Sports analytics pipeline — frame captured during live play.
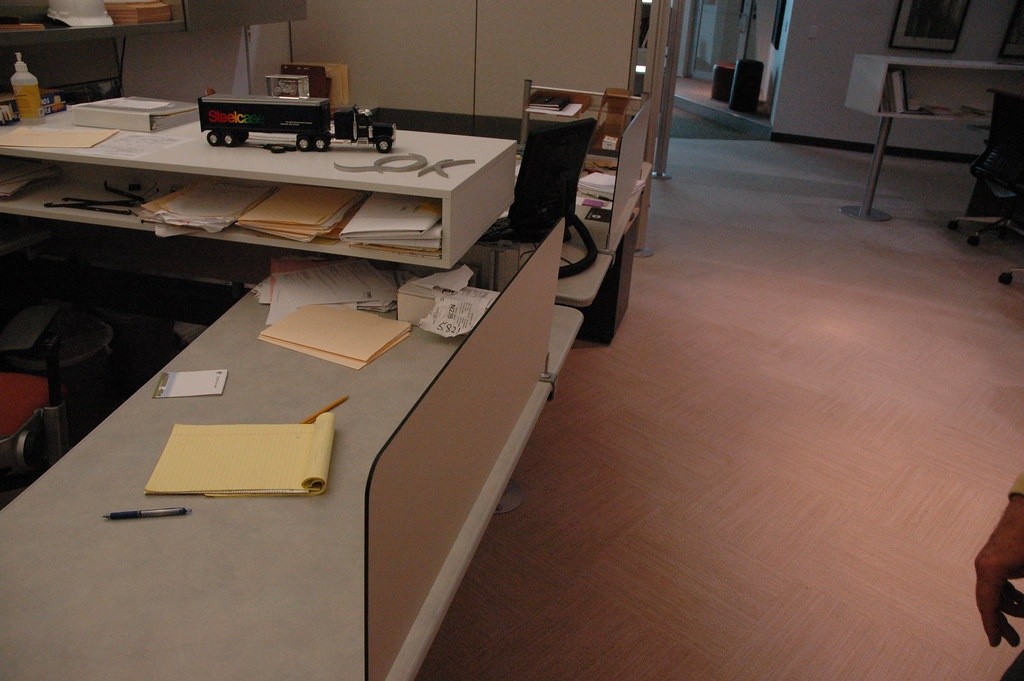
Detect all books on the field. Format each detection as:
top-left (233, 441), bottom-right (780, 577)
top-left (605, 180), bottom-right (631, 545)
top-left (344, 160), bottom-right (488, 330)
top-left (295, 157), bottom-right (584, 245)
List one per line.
top-left (529, 95), bottom-right (570, 110)
top-left (144, 411), bottom-right (335, 497)
top-left (880, 69), bottom-right (909, 113)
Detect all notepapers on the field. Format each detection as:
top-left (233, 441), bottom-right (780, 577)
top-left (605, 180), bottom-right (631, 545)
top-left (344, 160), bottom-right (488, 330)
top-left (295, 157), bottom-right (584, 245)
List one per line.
top-left (582, 199), bottom-right (603, 208)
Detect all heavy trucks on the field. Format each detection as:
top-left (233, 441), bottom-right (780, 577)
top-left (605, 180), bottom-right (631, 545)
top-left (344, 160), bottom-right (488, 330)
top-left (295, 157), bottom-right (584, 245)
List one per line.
top-left (197, 93), bottom-right (398, 152)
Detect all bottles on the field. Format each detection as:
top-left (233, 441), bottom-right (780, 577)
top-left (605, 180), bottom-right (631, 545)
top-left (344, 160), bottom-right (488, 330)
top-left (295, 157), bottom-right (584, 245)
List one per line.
top-left (11, 52), bottom-right (47, 126)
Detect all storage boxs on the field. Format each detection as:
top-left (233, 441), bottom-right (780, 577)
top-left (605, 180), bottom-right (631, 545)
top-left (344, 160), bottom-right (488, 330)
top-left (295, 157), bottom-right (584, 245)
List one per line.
top-left (398, 277), bottom-right (437, 325)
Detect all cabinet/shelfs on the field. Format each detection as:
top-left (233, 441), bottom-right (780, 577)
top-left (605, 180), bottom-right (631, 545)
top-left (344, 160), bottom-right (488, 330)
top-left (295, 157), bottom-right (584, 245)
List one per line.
top-left (0, 0), bottom-right (308, 48)
top-left (0, 101), bottom-right (514, 269)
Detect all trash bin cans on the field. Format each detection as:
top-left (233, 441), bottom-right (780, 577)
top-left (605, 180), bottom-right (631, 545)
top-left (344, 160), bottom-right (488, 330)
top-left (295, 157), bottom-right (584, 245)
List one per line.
top-left (728, 58), bottom-right (764, 113)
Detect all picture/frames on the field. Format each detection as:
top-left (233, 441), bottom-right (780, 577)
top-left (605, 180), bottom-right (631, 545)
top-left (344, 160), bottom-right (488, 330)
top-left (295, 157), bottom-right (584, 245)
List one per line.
top-left (770, 0), bottom-right (787, 49)
top-left (999, 0), bottom-right (1024, 57)
top-left (889, 0), bottom-right (970, 53)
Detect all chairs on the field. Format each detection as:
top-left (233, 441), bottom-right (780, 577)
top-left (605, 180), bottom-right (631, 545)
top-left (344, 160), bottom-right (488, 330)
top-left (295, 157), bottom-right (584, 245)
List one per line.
top-left (948, 89), bottom-right (1024, 245)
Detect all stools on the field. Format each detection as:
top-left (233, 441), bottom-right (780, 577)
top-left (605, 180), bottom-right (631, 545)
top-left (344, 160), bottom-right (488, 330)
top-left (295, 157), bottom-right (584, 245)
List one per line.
top-left (1, 311), bottom-right (130, 468)
top-left (0, 372), bottom-right (68, 475)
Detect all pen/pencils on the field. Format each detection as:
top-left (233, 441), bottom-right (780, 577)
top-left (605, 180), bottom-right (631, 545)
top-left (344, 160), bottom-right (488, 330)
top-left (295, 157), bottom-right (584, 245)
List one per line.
top-left (302, 393), bottom-right (350, 423)
top-left (581, 191), bottom-right (613, 202)
top-left (102, 507), bottom-right (192, 519)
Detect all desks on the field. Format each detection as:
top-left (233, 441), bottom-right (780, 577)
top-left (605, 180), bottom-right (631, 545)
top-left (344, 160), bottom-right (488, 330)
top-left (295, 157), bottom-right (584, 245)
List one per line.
top-left (839, 54), bottom-right (1024, 222)
top-left (0, 78), bottom-right (651, 681)
